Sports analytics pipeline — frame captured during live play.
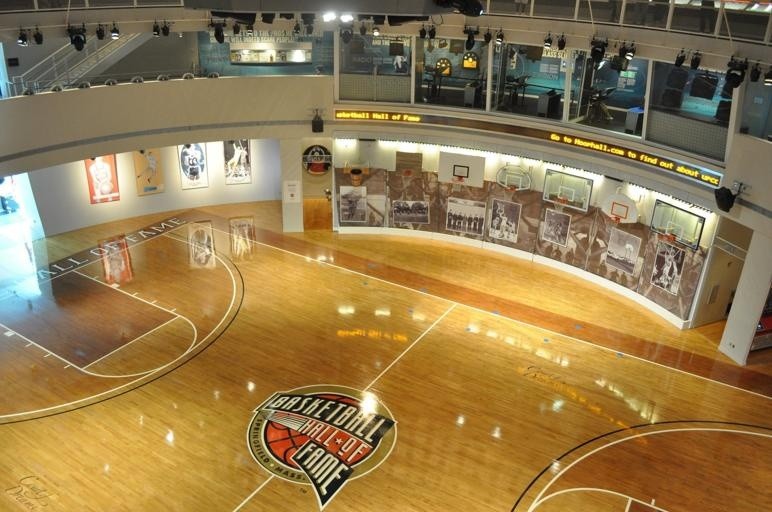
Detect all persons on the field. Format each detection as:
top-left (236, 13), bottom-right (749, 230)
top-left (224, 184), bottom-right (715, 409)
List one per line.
top-left (238, 226), bottom-right (253, 260)
top-left (88, 156), bottom-right (113, 202)
top-left (192, 230), bottom-right (211, 264)
top-left (226, 142), bottom-right (244, 178)
top-left (335, 171), bottom-right (704, 320)
top-left (102, 241), bottom-right (126, 284)
top-left (184, 143), bottom-right (202, 179)
top-left (238, 140), bottom-right (249, 177)
top-left (234, 227), bottom-right (244, 261)
top-left (137, 151), bottom-right (161, 185)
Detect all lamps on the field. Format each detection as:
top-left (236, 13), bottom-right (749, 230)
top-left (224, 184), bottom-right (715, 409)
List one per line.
top-left (260, 12), bottom-right (315, 36)
top-left (162, 20), bottom-right (171, 37)
top-left (94, 24), bottom-right (107, 42)
top-left (485, 28), bottom-right (492, 44)
top-left (543, 34), bottom-right (554, 49)
top-left (496, 27), bottom-right (504, 45)
top-left (151, 21), bottom-right (160, 37)
top-left (618, 40), bottom-right (637, 61)
top-left (33, 26), bottom-right (44, 45)
top-left (764, 66), bottom-right (772, 87)
top-left (556, 33), bottom-right (567, 51)
top-left (689, 50), bottom-right (703, 71)
top-left (389, 36), bottom-right (405, 58)
top-left (722, 55), bottom-right (747, 88)
top-left (463, 23), bottom-right (481, 51)
top-left (429, 24), bottom-right (437, 39)
top-left (749, 61), bottom-right (762, 82)
top-left (207, 10), bottom-right (257, 44)
top-left (323, 12), bottom-right (387, 45)
top-left (419, 21), bottom-right (427, 38)
top-left (66, 25), bottom-right (88, 52)
top-left (110, 22), bottom-right (121, 42)
top-left (688, 71), bottom-right (719, 101)
top-left (674, 48), bottom-right (690, 66)
top-left (18, 29), bottom-right (31, 48)
top-left (588, 35), bottom-right (609, 63)
